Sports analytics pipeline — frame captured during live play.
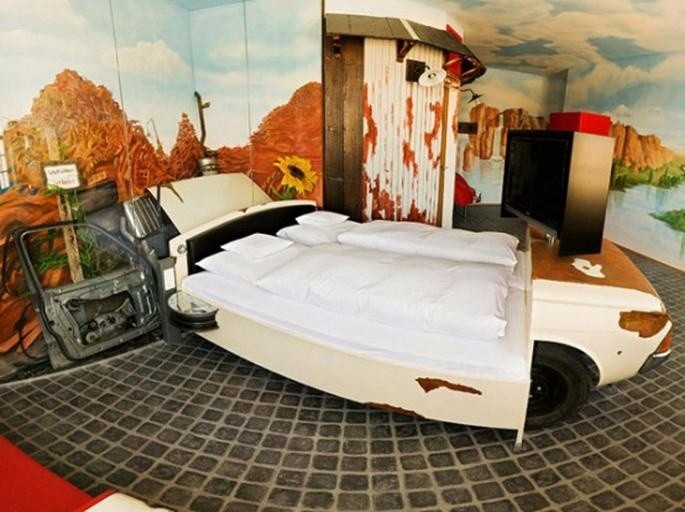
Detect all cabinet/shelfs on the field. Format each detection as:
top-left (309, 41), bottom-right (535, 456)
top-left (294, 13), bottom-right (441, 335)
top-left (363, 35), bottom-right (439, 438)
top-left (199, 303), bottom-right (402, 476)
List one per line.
top-left (498, 127), bottom-right (619, 260)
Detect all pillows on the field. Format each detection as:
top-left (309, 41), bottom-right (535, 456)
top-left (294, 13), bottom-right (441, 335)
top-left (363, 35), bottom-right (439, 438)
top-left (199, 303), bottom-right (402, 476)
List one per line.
top-left (295, 210), bottom-right (349, 227)
top-left (221, 232), bottom-right (293, 259)
top-left (276, 221), bottom-right (361, 248)
top-left (195, 240), bottom-right (310, 283)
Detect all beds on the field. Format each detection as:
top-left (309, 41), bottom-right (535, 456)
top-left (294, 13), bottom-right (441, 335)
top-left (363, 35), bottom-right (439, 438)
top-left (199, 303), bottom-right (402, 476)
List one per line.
top-left (168, 198), bottom-right (534, 452)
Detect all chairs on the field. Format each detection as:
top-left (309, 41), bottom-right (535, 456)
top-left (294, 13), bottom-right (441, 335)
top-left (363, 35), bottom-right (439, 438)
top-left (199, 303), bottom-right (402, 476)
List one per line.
top-left (454, 172), bottom-right (476, 217)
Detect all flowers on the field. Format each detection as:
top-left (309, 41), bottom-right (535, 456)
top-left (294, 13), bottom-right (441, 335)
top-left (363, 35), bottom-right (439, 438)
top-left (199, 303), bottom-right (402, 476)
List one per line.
top-left (270, 154), bottom-right (320, 200)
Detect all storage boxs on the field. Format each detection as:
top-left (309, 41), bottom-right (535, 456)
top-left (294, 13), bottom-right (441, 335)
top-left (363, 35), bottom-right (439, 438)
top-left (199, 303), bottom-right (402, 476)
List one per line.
top-left (549, 111), bottom-right (611, 138)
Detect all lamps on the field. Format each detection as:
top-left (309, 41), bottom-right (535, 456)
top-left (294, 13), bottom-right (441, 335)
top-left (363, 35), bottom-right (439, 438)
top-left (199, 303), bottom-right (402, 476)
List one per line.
top-left (453, 83), bottom-right (484, 105)
top-left (406, 58), bottom-right (447, 89)
